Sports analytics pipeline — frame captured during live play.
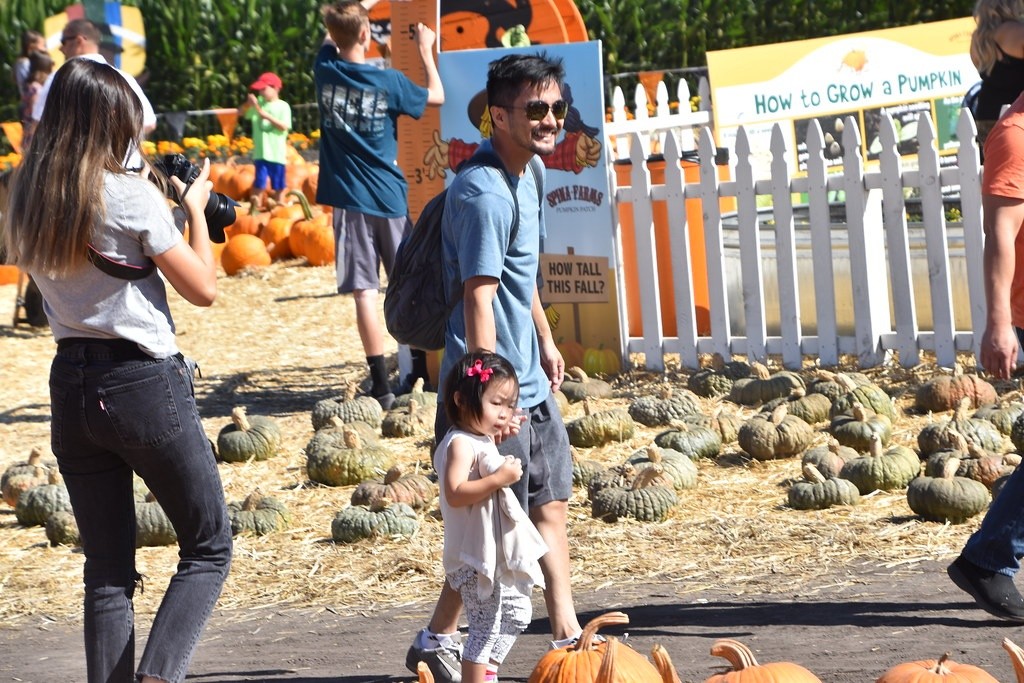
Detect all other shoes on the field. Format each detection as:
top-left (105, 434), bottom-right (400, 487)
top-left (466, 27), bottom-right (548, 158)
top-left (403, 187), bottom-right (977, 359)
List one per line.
top-left (367, 390), bottom-right (395, 411)
top-left (948, 557), bottom-right (1024, 623)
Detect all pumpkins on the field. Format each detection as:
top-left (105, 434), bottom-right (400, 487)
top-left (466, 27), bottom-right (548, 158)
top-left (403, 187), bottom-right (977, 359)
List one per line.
top-left (555, 361), bottom-right (1024, 522)
top-left (215, 376), bottom-right (439, 543)
top-left (0, 264), bottom-right (29, 286)
top-left (1, 447), bottom-right (178, 548)
top-left (526, 612), bottom-right (1024, 683)
top-left (163, 145), bottom-right (337, 275)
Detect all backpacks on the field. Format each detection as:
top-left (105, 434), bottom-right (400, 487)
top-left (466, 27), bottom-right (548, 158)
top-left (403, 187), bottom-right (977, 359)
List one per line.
top-left (384, 150), bottom-right (544, 351)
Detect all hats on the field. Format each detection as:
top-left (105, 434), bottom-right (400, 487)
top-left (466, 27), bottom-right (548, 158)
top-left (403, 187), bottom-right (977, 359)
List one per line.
top-left (251, 72), bottom-right (282, 90)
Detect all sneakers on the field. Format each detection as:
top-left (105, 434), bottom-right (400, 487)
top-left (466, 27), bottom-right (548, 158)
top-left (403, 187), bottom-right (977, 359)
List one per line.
top-left (405, 629), bottom-right (467, 683)
top-left (548, 630), bottom-right (648, 659)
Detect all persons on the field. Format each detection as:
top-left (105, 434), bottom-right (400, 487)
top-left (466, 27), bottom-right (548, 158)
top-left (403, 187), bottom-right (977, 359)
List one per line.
top-left (435, 347), bottom-right (532, 683)
top-left (239, 72), bottom-right (292, 205)
top-left (969, 0), bottom-right (1024, 140)
top-left (28, 19), bottom-right (156, 335)
top-left (17, 29), bottom-right (56, 153)
top-left (312, 5), bottom-right (445, 412)
top-left (402, 54), bottom-right (632, 683)
top-left (7, 59), bottom-right (232, 683)
top-left (945, 91), bottom-right (1023, 621)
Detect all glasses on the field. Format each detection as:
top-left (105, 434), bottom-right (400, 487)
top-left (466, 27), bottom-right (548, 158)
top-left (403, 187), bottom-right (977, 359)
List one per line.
top-left (60, 34), bottom-right (88, 42)
top-left (500, 98), bottom-right (569, 121)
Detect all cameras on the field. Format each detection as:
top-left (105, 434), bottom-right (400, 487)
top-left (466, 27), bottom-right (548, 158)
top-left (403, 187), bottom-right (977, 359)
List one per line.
top-left (146, 153), bottom-right (241, 244)
